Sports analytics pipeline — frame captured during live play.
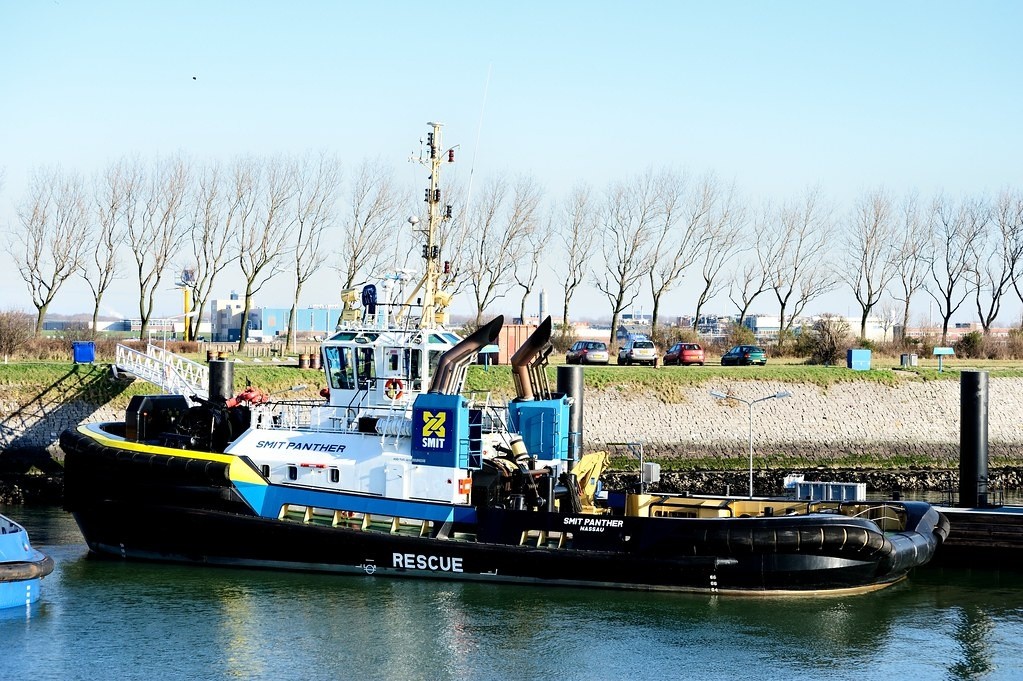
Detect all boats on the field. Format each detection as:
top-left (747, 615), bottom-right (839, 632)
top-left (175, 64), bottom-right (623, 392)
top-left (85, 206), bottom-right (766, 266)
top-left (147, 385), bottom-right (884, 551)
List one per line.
top-left (59, 119), bottom-right (951, 600)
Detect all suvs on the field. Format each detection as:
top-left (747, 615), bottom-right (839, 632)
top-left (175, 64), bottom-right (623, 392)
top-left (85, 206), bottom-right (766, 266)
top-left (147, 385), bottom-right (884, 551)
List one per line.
top-left (617, 339), bottom-right (659, 366)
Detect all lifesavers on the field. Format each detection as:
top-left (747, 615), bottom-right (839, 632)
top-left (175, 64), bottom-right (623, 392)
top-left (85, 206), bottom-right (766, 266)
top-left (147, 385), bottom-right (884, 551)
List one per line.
top-left (385, 379), bottom-right (405, 400)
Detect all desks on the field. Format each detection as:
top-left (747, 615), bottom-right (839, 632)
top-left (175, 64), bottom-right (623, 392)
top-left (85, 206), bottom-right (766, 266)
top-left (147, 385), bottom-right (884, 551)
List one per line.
top-left (796, 481), bottom-right (867, 502)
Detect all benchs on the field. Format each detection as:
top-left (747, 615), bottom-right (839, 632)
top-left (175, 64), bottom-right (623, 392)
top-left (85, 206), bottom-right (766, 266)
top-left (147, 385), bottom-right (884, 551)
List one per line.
top-left (940, 479), bottom-right (959, 507)
top-left (977, 480), bottom-right (1004, 509)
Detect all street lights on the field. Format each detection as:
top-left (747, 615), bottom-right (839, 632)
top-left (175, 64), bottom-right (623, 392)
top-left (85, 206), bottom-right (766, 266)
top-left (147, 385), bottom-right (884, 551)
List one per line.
top-left (708, 390), bottom-right (792, 501)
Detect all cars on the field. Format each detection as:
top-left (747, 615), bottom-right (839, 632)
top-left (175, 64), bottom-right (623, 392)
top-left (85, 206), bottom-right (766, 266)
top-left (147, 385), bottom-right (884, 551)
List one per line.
top-left (721, 345), bottom-right (767, 366)
top-left (566, 341), bottom-right (609, 365)
top-left (663, 343), bottom-right (705, 367)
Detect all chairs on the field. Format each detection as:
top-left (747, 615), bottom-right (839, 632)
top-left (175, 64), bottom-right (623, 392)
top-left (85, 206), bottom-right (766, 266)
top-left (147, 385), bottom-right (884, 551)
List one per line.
top-left (639, 344), bottom-right (643, 347)
